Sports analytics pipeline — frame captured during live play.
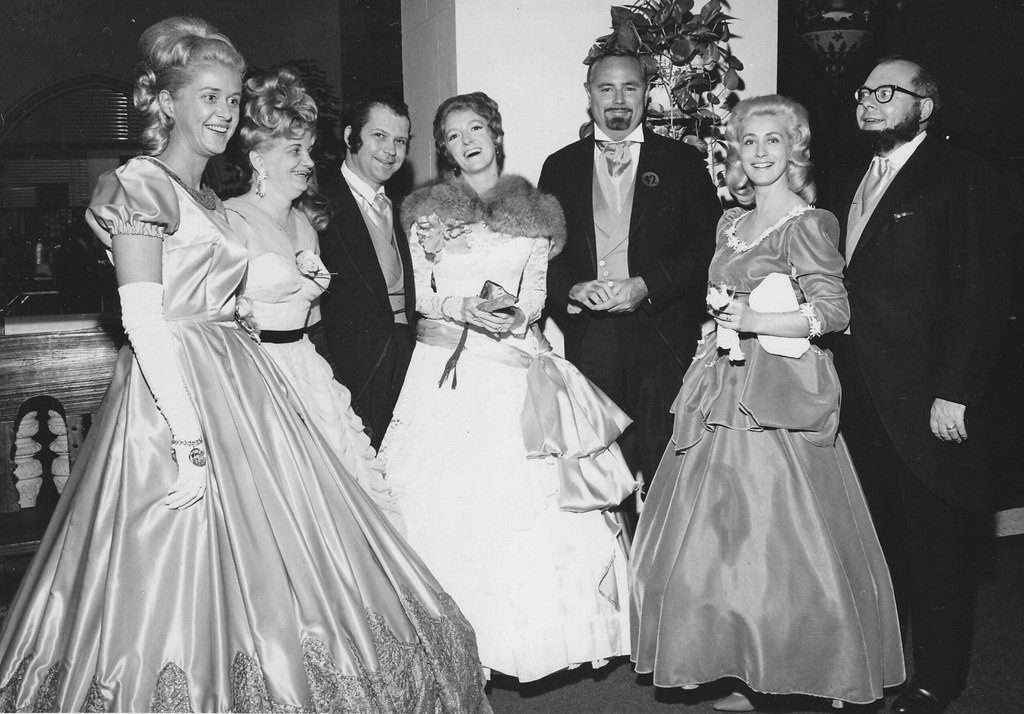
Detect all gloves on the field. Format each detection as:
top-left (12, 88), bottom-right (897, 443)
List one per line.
top-left (304, 319), bottom-right (372, 440)
top-left (118, 283), bottom-right (206, 510)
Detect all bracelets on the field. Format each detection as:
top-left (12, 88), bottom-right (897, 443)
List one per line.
top-left (170, 435), bottom-right (208, 468)
top-left (440, 295), bottom-right (456, 322)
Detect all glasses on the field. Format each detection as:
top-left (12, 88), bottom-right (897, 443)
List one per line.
top-left (855, 85), bottom-right (926, 105)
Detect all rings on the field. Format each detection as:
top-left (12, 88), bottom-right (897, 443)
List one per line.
top-left (472, 316), bottom-right (477, 321)
top-left (724, 315), bottom-right (731, 321)
top-left (947, 424), bottom-right (956, 430)
top-left (498, 324), bottom-right (503, 331)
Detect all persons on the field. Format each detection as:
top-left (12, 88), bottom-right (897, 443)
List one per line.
top-left (201, 65), bottom-right (409, 562)
top-left (293, 88), bottom-right (418, 451)
top-left (369, 91), bottom-right (636, 687)
top-left (823, 56), bottom-right (989, 714)
top-left (534, 50), bottom-right (720, 561)
top-left (81, 13), bottom-right (300, 714)
top-left (622, 94), bottom-right (907, 711)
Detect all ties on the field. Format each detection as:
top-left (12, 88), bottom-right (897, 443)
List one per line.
top-left (346, 179), bottom-right (392, 244)
top-left (862, 159), bottom-right (892, 215)
top-left (596, 140), bottom-right (635, 214)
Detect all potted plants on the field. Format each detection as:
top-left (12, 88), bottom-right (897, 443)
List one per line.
top-left (580, 0), bottom-right (743, 212)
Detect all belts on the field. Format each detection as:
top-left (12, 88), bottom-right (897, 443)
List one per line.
top-left (259, 328), bottom-right (304, 344)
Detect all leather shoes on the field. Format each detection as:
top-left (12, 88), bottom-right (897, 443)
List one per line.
top-left (891, 676), bottom-right (952, 714)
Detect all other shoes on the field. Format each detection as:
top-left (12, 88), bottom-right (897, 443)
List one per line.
top-left (714, 692), bottom-right (755, 712)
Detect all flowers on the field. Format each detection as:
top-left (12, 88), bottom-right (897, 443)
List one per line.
top-left (294, 249), bottom-right (336, 294)
top-left (414, 213), bottom-right (472, 265)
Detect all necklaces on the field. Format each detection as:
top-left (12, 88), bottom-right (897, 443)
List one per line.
top-left (479, 220), bottom-right (488, 228)
